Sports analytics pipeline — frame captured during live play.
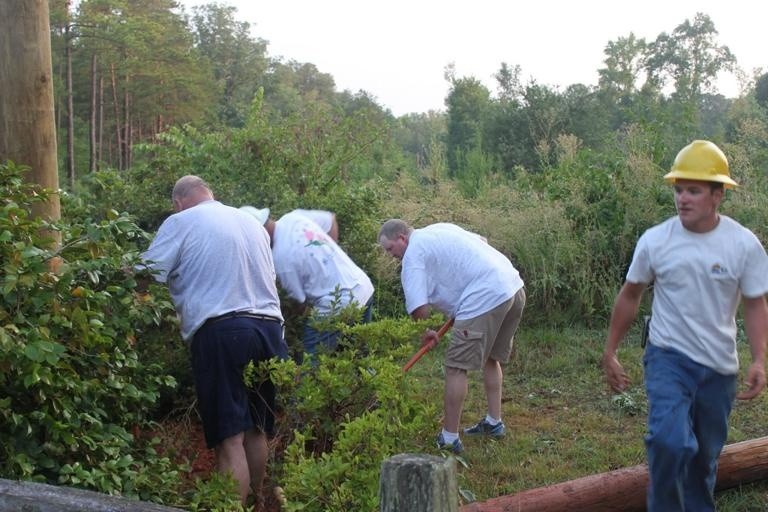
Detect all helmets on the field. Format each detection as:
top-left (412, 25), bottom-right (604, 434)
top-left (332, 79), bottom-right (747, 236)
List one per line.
top-left (663, 139), bottom-right (739, 190)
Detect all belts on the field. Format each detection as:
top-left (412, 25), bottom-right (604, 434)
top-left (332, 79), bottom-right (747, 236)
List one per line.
top-left (209, 310), bottom-right (281, 323)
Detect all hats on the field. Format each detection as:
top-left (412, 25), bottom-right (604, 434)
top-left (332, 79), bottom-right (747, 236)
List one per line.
top-left (239, 206), bottom-right (270, 225)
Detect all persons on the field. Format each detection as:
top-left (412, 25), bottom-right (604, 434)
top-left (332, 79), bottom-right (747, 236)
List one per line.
top-left (602, 140), bottom-right (767, 510)
top-left (377, 219), bottom-right (527, 452)
top-left (121, 174), bottom-right (290, 510)
top-left (239, 206), bottom-right (375, 451)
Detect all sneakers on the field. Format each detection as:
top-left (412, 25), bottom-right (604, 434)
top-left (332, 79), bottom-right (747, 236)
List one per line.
top-left (434, 432), bottom-right (463, 455)
top-left (464, 419), bottom-right (505, 437)
